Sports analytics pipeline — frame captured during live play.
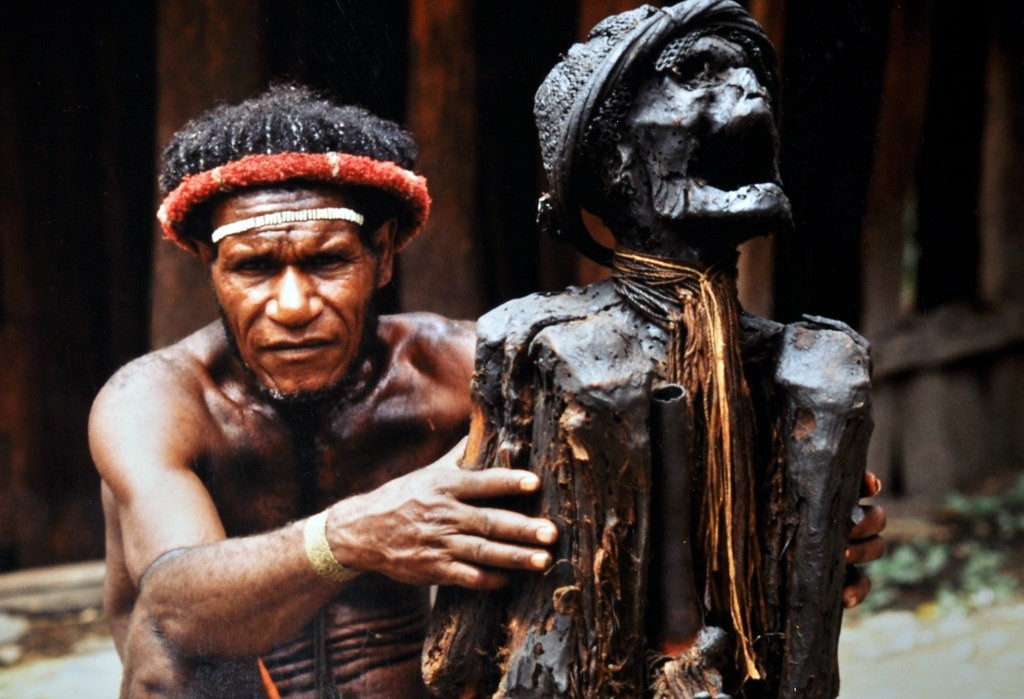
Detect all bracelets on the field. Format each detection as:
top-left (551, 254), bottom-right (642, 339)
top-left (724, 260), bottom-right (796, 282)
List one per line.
top-left (301, 504), bottom-right (359, 587)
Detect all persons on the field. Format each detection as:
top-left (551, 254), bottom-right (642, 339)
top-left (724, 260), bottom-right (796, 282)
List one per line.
top-left (86, 78), bottom-right (888, 699)
top-left (411, 0), bottom-right (877, 699)
